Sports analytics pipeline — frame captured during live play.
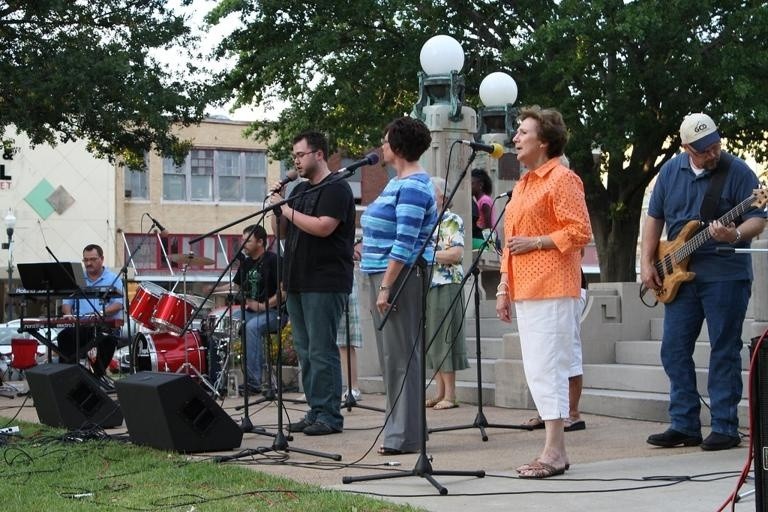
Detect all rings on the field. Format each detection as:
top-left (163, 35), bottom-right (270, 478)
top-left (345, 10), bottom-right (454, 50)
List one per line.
top-left (498, 315), bottom-right (501, 318)
top-left (714, 233), bottom-right (717, 236)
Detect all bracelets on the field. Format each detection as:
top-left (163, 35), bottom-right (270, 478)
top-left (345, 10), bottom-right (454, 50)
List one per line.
top-left (496, 282), bottom-right (510, 289)
top-left (731, 230), bottom-right (742, 246)
top-left (496, 292), bottom-right (508, 296)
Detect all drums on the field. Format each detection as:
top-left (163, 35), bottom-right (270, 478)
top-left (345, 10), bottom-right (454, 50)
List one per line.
top-left (129, 282), bottom-right (166, 331)
top-left (208, 315), bottom-right (237, 337)
top-left (149, 291), bottom-right (192, 333)
top-left (131, 332), bottom-right (205, 374)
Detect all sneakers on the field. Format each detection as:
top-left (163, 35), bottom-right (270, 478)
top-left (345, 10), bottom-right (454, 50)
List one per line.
top-left (302, 421), bottom-right (343, 436)
top-left (285, 419), bottom-right (315, 432)
top-left (342, 389), bottom-right (361, 401)
top-left (236, 383), bottom-right (263, 396)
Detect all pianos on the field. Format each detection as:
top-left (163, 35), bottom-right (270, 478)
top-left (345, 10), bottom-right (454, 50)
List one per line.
top-left (8, 286), bottom-right (123, 300)
top-left (22, 317), bottom-right (123, 331)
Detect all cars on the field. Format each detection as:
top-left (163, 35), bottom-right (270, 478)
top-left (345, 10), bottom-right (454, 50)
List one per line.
top-left (0, 320), bottom-right (60, 380)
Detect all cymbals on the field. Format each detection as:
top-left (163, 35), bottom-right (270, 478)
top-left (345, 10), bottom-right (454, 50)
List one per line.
top-left (166, 252), bottom-right (214, 268)
top-left (214, 281), bottom-right (244, 293)
top-left (180, 292), bottom-right (213, 311)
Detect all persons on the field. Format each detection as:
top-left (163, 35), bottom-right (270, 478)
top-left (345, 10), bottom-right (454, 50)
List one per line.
top-left (360, 117), bottom-right (437, 456)
top-left (58, 244), bottom-right (126, 379)
top-left (640, 113), bottom-right (767, 450)
top-left (202, 224), bottom-right (289, 394)
top-left (268, 132), bottom-right (355, 435)
top-left (471, 168), bottom-right (498, 239)
top-left (495, 104), bottom-right (592, 478)
top-left (426, 177), bottom-right (464, 410)
top-left (521, 154), bottom-right (586, 432)
top-left (296, 225), bottom-right (362, 402)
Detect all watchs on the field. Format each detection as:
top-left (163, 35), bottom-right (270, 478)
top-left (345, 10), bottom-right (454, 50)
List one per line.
top-left (377, 285), bottom-right (388, 290)
top-left (536, 238), bottom-right (542, 249)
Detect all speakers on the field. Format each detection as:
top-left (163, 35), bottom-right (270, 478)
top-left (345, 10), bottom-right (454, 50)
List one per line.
top-left (113, 369), bottom-right (243, 452)
top-left (23, 361), bottom-right (122, 431)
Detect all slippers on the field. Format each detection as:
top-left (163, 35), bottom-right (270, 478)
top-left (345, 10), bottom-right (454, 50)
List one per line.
top-left (516, 458), bottom-right (570, 473)
top-left (561, 418), bottom-right (586, 432)
top-left (518, 460), bottom-right (566, 479)
top-left (522, 416), bottom-right (545, 429)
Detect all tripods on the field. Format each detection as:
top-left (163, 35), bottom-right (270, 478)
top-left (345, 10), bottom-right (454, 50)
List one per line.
top-left (237, 311), bottom-right (308, 410)
top-left (342, 272), bottom-right (484, 495)
top-left (429, 279), bottom-right (534, 440)
top-left (212, 219), bottom-right (341, 464)
top-left (341, 302), bottom-right (386, 413)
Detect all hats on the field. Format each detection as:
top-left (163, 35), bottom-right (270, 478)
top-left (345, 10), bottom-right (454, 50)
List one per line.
top-left (680, 113), bottom-right (721, 154)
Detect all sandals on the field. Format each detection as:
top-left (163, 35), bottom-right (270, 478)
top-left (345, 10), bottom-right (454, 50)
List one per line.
top-left (377, 446), bottom-right (420, 455)
top-left (433, 399), bottom-right (459, 410)
top-left (422, 397), bottom-right (443, 407)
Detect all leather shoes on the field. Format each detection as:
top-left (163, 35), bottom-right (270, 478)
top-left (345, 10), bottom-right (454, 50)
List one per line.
top-left (700, 432), bottom-right (741, 451)
top-left (646, 429), bottom-right (702, 449)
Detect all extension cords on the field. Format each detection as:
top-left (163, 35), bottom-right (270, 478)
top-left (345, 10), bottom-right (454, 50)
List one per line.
top-left (0, 426), bottom-right (21, 435)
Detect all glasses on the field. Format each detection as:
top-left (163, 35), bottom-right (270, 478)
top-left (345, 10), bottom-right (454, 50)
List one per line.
top-left (81, 257), bottom-right (98, 262)
top-left (380, 139), bottom-right (389, 145)
top-left (696, 144), bottom-right (721, 158)
top-left (290, 149), bottom-right (320, 160)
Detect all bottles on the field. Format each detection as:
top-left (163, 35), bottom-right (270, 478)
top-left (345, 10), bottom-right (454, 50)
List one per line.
top-left (228, 368), bottom-right (239, 399)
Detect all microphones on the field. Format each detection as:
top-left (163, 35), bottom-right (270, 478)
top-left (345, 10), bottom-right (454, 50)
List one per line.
top-left (457, 133), bottom-right (506, 165)
top-left (149, 210), bottom-right (170, 241)
top-left (340, 152), bottom-right (385, 176)
top-left (273, 167), bottom-right (298, 193)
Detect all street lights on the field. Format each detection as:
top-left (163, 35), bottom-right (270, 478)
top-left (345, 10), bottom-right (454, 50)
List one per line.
top-left (3, 208), bottom-right (16, 326)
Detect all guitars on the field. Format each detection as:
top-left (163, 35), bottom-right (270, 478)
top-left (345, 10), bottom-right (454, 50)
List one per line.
top-left (643, 184), bottom-right (768, 303)
